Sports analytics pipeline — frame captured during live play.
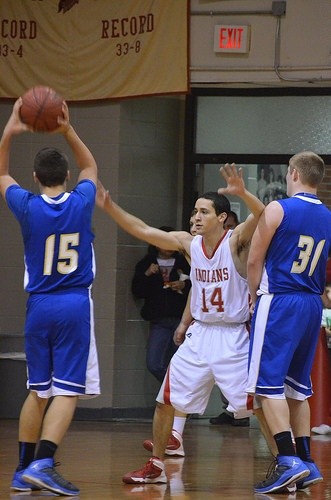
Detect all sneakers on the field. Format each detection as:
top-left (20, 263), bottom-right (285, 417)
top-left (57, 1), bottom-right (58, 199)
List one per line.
top-left (311, 424), bottom-right (331, 434)
top-left (143, 429), bottom-right (186, 456)
top-left (21, 458), bottom-right (80, 495)
top-left (296, 459), bottom-right (324, 490)
top-left (252, 455), bottom-right (312, 494)
top-left (285, 483), bottom-right (297, 492)
top-left (122, 457), bottom-right (167, 483)
top-left (11, 468), bottom-right (47, 491)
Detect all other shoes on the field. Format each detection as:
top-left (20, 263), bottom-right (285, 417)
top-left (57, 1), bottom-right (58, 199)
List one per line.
top-left (209, 412), bottom-right (232, 424)
top-left (232, 418), bottom-right (250, 426)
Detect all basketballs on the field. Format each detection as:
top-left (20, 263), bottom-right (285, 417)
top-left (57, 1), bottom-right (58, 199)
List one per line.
top-left (17, 85), bottom-right (68, 133)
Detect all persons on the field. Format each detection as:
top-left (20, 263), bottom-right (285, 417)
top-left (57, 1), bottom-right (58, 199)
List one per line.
top-left (0, 97), bottom-right (98, 496)
top-left (311, 282), bottom-right (331, 436)
top-left (95, 163), bottom-right (297, 492)
top-left (131, 211), bottom-right (251, 456)
top-left (246, 152), bottom-right (331, 494)
